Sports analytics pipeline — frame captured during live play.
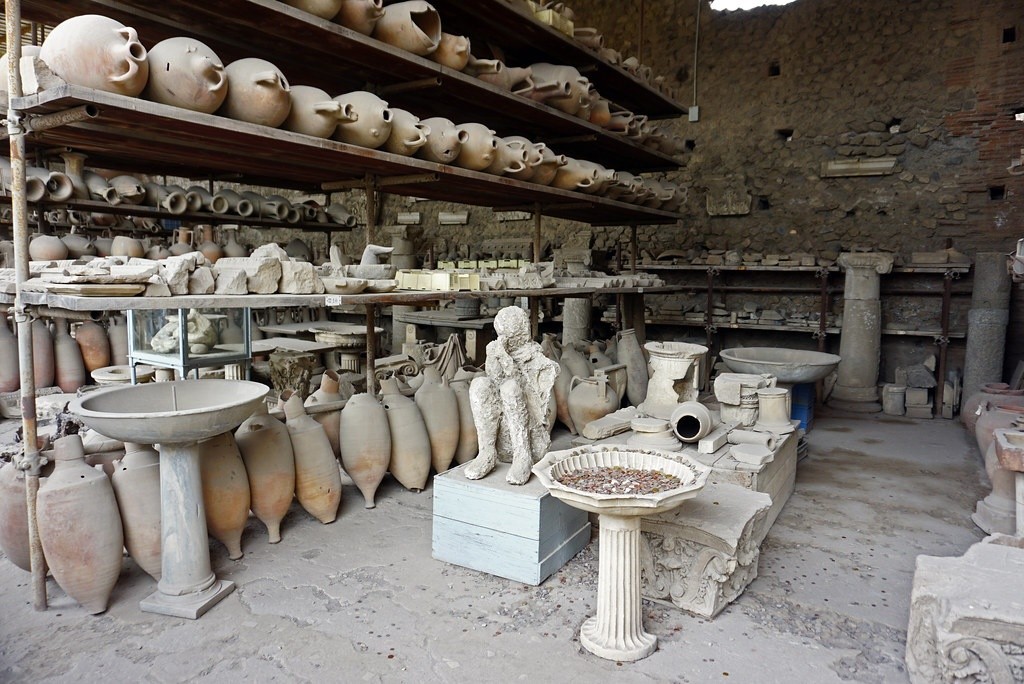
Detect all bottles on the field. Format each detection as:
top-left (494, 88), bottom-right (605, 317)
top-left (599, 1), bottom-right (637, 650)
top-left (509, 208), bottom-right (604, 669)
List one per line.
top-left (962, 384), bottom-right (1024, 485)
top-left (0, 156), bottom-right (357, 232)
top-left (0, 14), bottom-right (689, 213)
top-left (513, 0), bottom-right (680, 100)
top-left (283, 0), bottom-right (689, 156)
top-left (0, 314), bottom-right (128, 394)
top-left (0, 224), bottom-right (329, 269)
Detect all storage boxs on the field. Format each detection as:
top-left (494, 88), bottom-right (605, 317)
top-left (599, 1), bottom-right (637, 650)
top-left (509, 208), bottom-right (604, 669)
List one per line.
top-left (125, 306), bottom-right (252, 366)
top-left (430, 458), bottom-right (593, 587)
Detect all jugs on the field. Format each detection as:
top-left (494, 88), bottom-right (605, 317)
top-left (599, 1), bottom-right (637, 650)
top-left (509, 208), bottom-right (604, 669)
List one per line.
top-left (219, 307), bottom-right (328, 344)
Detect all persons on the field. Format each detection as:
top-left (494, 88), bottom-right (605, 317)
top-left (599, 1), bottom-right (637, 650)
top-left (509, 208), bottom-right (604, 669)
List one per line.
top-left (463, 306), bottom-right (562, 484)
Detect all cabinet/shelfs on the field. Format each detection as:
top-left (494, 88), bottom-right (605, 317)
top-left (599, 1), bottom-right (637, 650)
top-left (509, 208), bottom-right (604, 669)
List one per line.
top-left (0, 0), bottom-right (704, 609)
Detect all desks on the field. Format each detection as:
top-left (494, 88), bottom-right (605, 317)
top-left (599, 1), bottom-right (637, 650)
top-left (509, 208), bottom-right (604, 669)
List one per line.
top-left (396, 310), bottom-right (494, 371)
top-left (126, 354), bottom-right (253, 386)
